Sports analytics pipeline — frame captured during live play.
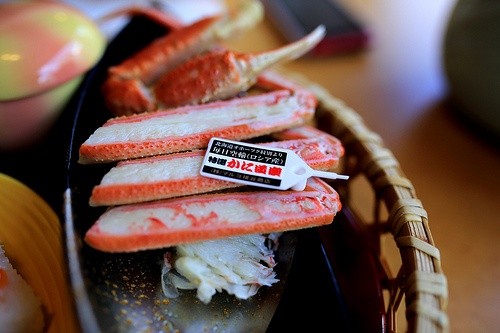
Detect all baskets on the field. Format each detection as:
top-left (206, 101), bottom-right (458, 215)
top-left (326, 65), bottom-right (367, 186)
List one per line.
top-left (283, 66), bottom-right (451, 333)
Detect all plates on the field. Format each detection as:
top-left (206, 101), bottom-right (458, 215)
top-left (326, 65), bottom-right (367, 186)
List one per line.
top-left (65, 11), bottom-right (354, 333)
top-left (0, 172), bottom-right (80, 333)
